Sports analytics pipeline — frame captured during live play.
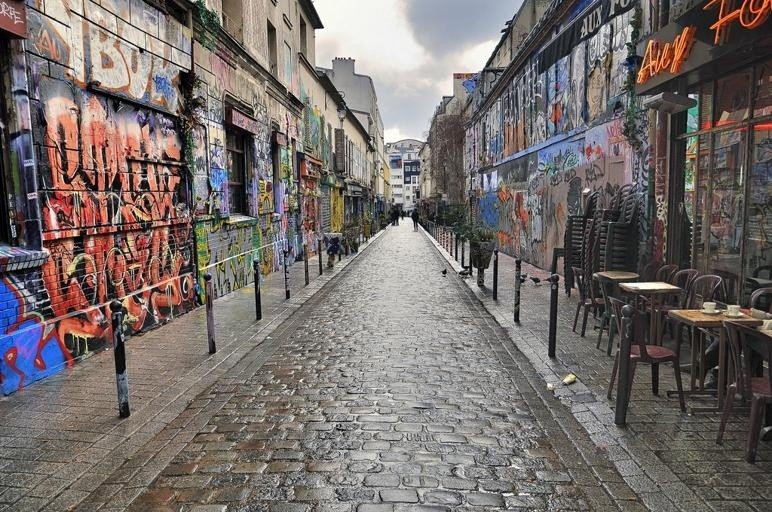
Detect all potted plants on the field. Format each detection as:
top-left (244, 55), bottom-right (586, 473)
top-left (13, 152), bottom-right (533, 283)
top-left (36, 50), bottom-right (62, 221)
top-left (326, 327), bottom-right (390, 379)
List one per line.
top-left (464, 222), bottom-right (496, 270)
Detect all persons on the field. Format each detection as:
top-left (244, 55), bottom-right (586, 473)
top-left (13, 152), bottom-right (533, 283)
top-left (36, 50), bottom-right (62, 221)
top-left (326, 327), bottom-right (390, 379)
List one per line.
top-left (400, 209), bottom-right (405, 221)
top-left (411, 207), bottom-right (421, 231)
top-left (407, 209), bottom-right (409, 216)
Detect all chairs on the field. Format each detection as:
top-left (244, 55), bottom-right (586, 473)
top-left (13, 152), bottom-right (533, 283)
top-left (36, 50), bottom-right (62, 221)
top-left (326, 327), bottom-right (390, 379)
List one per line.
top-left (550, 184), bottom-right (650, 299)
top-left (604, 295), bottom-right (687, 415)
top-left (714, 320), bottom-right (771, 465)
top-left (571, 263), bottom-right (724, 356)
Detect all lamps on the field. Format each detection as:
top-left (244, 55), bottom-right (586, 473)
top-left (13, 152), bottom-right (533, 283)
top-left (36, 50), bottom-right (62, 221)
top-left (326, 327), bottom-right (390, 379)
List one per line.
top-left (324, 90), bottom-right (347, 127)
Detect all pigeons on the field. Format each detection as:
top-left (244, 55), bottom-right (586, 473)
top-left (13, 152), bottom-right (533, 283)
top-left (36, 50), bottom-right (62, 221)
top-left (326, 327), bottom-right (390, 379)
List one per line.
top-left (529, 276), bottom-right (541, 285)
top-left (442, 267), bottom-right (447, 276)
top-left (520, 272), bottom-right (528, 278)
top-left (458, 270), bottom-right (473, 276)
top-left (542, 273), bottom-right (559, 282)
top-left (520, 279), bottom-right (526, 284)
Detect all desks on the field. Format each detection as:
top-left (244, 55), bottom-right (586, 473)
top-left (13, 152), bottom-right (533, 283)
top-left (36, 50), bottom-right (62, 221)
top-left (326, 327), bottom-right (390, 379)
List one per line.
top-left (666, 307), bottom-right (771, 414)
top-left (617, 281), bottom-right (683, 342)
top-left (591, 269), bottom-right (642, 283)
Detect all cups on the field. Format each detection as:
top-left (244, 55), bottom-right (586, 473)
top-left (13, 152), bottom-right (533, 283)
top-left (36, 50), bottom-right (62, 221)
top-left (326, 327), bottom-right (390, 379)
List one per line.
top-left (702, 302), bottom-right (717, 313)
top-left (727, 304), bottom-right (742, 315)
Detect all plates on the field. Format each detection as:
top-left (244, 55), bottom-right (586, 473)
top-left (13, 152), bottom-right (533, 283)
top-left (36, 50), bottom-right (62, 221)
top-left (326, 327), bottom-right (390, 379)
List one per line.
top-left (724, 312), bottom-right (745, 319)
top-left (700, 310), bottom-right (722, 314)
